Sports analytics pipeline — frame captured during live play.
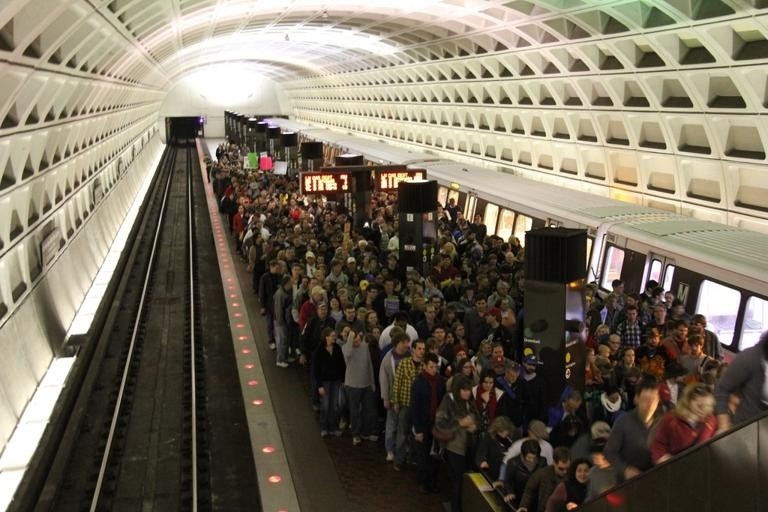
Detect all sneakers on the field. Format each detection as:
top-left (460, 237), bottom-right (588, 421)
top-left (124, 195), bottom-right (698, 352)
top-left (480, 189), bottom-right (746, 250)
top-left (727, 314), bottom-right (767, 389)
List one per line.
top-left (269, 343), bottom-right (301, 368)
top-left (321, 419), bottom-right (379, 446)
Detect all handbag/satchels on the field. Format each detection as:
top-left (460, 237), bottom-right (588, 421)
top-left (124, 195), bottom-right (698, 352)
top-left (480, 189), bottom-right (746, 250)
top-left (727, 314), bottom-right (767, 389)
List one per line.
top-left (433, 427), bottom-right (455, 441)
top-left (429, 443), bottom-right (447, 463)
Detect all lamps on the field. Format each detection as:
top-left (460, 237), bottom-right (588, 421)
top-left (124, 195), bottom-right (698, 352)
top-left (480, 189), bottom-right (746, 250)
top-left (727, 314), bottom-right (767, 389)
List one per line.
top-left (301, 142), bottom-right (323, 160)
top-left (522, 227), bottom-right (587, 287)
top-left (222, 110), bottom-right (281, 142)
top-left (280, 132), bottom-right (298, 148)
top-left (335, 153), bottom-right (364, 167)
top-left (397, 179), bottom-right (438, 213)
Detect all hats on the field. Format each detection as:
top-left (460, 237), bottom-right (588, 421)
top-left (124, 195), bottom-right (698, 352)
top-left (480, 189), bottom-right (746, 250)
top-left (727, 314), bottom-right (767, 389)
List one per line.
top-left (530, 421), bottom-right (550, 439)
top-left (525, 354), bottom-right (538, 365)
top-left (305, 252), bottom-right (315, 260)
top-left (591, 421), bottom-right (610, 441)
top-left (643, 328), bottom-right (662, 337)
top-left (360, 280), bottom-right (369, 290)
top-left (346, 257), bottom-right (356, 265)
